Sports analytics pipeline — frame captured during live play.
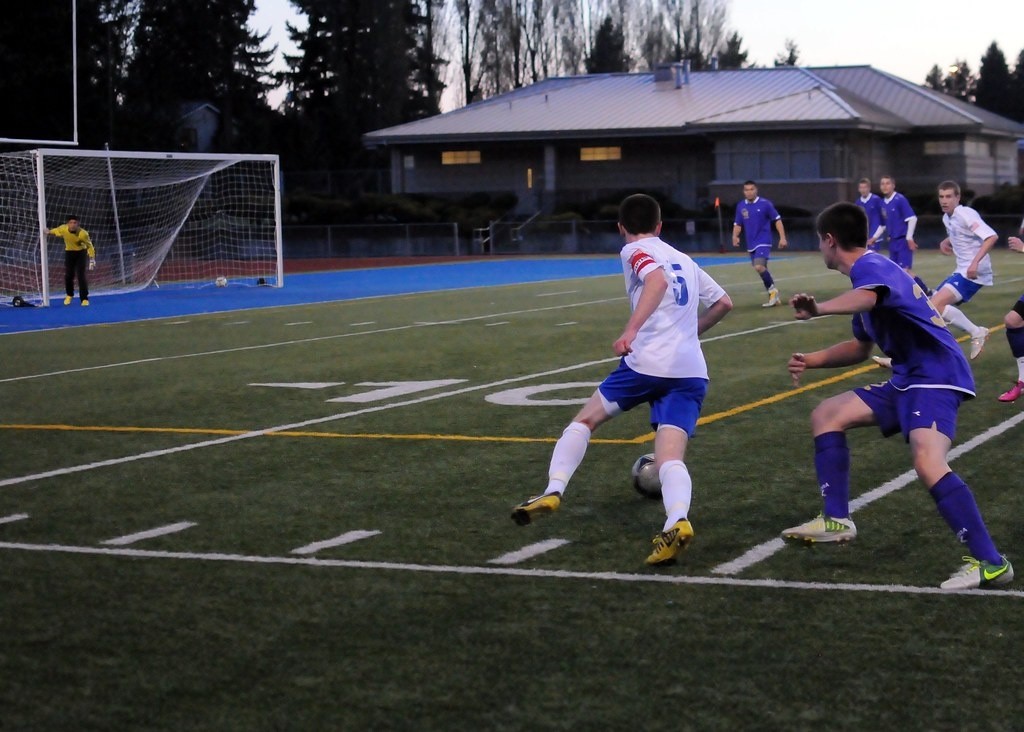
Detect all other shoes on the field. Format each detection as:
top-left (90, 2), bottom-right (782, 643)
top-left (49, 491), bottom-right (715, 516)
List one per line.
top-left (768, 284), bottom-right (779, 306)
top-left (64, 295), bottom-right (72, 305)
top-left (81, 299), bottom-right (89, 306)
top-left (762, 303), bottom-right (769, 307)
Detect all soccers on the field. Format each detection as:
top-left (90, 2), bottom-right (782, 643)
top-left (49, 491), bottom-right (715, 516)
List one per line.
top-left (631, 452), bottom-right (662, 499)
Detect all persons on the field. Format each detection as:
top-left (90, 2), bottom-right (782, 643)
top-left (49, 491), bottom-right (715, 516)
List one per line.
top-left (43, 215), bottom-right (97, 306)
top-left (867, 176), bottom-right (918, 273)
top-left (732, 181), bottom-right (789, 308)
top-left (511, 194), bottom-right (733, 565)
top-left (854, 177), bottom-right (884, 252)
top-left (931, 180), bottom-right (999, 360)
top-left (998, 234), bottom-right (1024, 402)
top-left (780, 201), bottom-right (1014, 592)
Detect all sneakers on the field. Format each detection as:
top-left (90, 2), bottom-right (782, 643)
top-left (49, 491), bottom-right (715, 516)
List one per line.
top-left (970, 326), bottom-right (991, 360)
top-left (511, 491), bottom-right (562, 526)
top-left (781, 510), bottom-right (857, 547)
top-left (998, 380), bottom-right (1024, 402)
top-left (871, 356), bottom-right (893, 369)
top-left (940, 556), bottom-right (1014, 590)
top-left (644, 518), bottom-right (694, 567)
